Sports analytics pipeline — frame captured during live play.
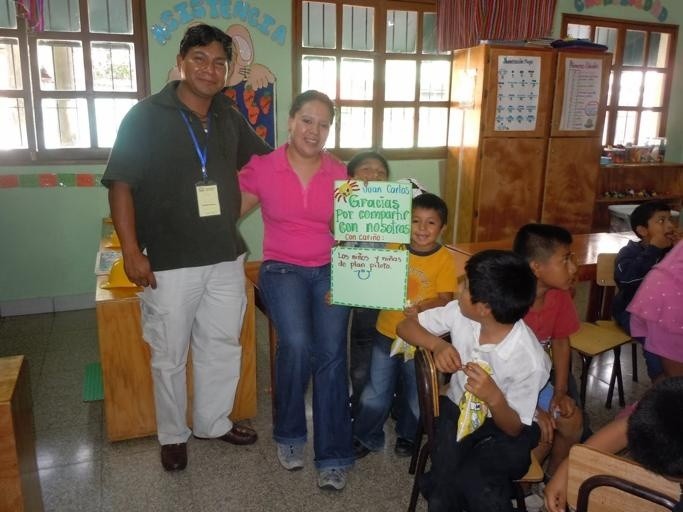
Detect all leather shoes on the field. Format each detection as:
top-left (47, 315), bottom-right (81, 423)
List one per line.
top-left (395, 435), bottom-right (413, 457)
top-left (191, 423), bottom-right (257, 445)
top-left (162, 443), bottom-right (188, 472)
top-left (350, 437), bottom-right (370, 459)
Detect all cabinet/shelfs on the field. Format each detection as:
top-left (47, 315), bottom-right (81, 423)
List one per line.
top-left (442, 37), bottom-right (613, 242)
top-left (594, 160), bottom-right (683, 232)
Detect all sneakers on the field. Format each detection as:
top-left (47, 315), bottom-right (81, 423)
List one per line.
top-left (278, 442), bottom-right (305, 469)
top-left (319, 467), bottom-right (346, 489)
top-left (390, 398), bottom-right (402, 420)
top-left (512, 471), bottom-right (550, 512)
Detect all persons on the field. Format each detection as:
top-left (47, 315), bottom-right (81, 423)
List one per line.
top-left (511, 221), bottom-right (585, 500)
top-left (613, 202), bottom-right (677, 384)
top-left (395, 247), bottom-right (554, 511)
top-left (353, 192), bottom-right (460, 456)
top-left (542, 373), bottom-right (683, 511)
top-left (237, 88), bottom-right (357, 493)
top-left (99, 22), bottom-right (277, 472)
top-left (625, 233), bottom-right (683, 378)
top-left (343, 152), bottom-right (391, 420)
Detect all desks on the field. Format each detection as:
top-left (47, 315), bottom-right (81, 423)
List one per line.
top-left (95, 236), bottom-right (259, 443)
top-left (440, 232), bottom-right (643, 323)
top-left (244, 260), bottom-right (278, 439)
top-left (0, 355), bottom-right (45, 512)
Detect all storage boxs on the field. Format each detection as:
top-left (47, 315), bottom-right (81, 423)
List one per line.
top-left (607, 204), bottom-right (680, 232)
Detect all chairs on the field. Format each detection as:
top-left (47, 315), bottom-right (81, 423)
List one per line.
top-left (568, 252), bottom-right (647, 409)
top-left (407, 347), bottom-right (544, 512)
top-left (567, 443), bottom-right (682, 512)
